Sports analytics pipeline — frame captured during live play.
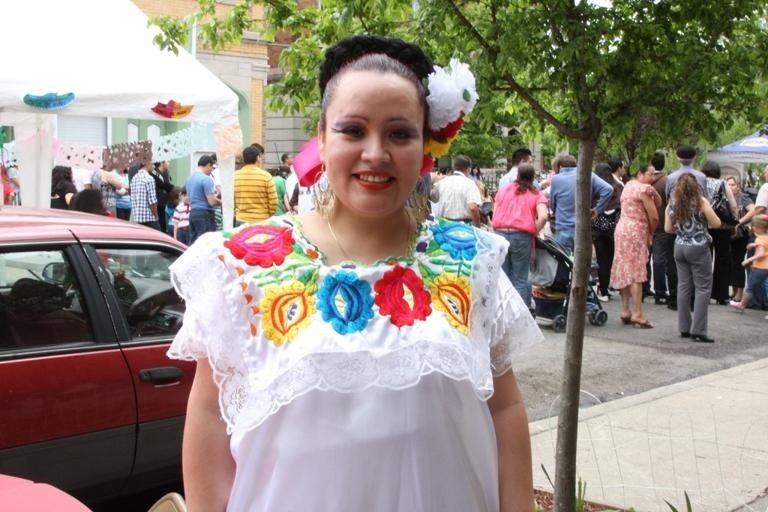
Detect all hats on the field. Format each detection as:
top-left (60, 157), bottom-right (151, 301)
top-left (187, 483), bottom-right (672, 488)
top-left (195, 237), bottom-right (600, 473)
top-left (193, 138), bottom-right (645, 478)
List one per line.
top-left (676, 145), bottom-right (696, 163)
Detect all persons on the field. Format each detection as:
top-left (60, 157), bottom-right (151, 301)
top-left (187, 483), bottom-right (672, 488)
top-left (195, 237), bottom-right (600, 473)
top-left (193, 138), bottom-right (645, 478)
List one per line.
top-left (51, 165), bottom-right (78, 210)
top-left (607, 159), bottom-right (625, 187)
top-left (430, 154), bottom-right (482, 230)
top-left (281, 153), bottom-right (293, 165)
top-left (172, 184), bottom-right (191, 245)
top-left (431, 155), bottom-right (452, 185)
top-left (0, 160), bottom-right (15, 206)
top-left (251, 143), bottom-right (264, 155)
top-left (162, 160), bottom-right (170, 183)
top-left (112, 167), bottom-right (131, 221)
top-left (129, 162), bottom-right (161, 231)
top-left (665, 145), bottom-right (707, 312)
top-left (91, 167), bottom-right (122, 219)
top-left (724, 174), bottom-right (755, 301)
top-left (468, 164), bottom-right (482, 183)
top-left (538, 157), bottom-right (555, 196)
top-left (68, 189), bottom-right (116, 218)
top-left (701, 160), bottom-right (738, 304)
top-left (663, 172), bottom-right (722, 342)
top-left (729, 213), bottom-right (768, 319)
top-left (271, 166), bottom-right (291, 216)
top-left (164, 188), bottom-right (181, 234)
top-left (153, 160), bottom-right (164, 183)
top-left (209, 154), bottom-right (221, 193)
top-left (608, 163), bottom-right (662, 330)
top-left (185, 155), bottom-right (222, 246)
top-left (592, 162), bottom-right (624, 301)
top-left (642, 151), bottom-right (669, 305)
top-left (128, 166), bottom-right (174, 210)
top-left (5, 145), bottom-right (21, 206)
top-left (497, 148), bottom-right (540, 193)
top-left (166, 33), bottom-right (545, 511)
top-left (549, 153), bottom-right (614, 259)
top-left (214, 192), bottom-right (223, 231)
top-left (289, 172), bottom-right (328, 216)
top-left (491, 163), bottom-right (549, 308)
top-left (746, 165), bottom-right (768, 309)
top-left (233, 145), bottom-right (278, 228)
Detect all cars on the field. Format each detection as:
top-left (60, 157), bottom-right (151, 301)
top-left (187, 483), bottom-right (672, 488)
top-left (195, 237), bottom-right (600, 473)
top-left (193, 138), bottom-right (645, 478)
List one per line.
top-left (0, 205), bottom-right (217, 492)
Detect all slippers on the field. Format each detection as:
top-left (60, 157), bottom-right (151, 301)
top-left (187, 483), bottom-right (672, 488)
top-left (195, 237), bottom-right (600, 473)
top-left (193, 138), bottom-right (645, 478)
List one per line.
top-left (631, 320), bottom-right (656, 329)
top-left (621, 314), bottom-right (631, 325)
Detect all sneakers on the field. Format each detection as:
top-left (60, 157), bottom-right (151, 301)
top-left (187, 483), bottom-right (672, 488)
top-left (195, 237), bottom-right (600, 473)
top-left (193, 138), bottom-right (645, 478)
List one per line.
top-left (596, 294), bottom-right (609, 301)
top-left (730, 300), bottom-right (746, 312)
top-left (652, 288), bottom-right (679, 313)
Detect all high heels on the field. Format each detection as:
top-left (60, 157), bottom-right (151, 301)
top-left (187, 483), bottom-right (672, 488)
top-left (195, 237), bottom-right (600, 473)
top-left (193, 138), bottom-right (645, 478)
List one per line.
top-left (692, 334), bottom-right (716, 343)
top-left (682, 331), bottom-right (692, 339)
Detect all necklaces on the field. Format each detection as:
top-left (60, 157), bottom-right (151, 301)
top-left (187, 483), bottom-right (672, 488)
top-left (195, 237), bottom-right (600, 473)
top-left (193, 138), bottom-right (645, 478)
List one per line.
top-left (321, 208), bottom-right (413, 264)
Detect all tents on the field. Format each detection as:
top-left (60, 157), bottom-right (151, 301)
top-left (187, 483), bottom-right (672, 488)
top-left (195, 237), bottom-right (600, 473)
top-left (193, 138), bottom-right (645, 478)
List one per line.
top-left (1, 0), bottom-right (244, 243)
top-left (706, 126), bottom-right (768, 178)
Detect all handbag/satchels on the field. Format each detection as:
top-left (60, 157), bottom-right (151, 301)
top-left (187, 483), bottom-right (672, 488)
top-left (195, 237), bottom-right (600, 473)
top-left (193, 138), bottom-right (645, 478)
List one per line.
top-left (710, 185), bottom-right (740, 227)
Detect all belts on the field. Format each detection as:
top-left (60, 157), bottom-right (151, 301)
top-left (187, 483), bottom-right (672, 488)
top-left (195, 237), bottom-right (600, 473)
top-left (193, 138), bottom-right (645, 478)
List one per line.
top-left (497, 228), bottom-right (519, 232)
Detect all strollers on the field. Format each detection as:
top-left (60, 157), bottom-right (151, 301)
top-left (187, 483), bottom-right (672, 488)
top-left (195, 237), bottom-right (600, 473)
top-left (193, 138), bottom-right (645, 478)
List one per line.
top-left (528, 231), bottom-right (607, 332)
